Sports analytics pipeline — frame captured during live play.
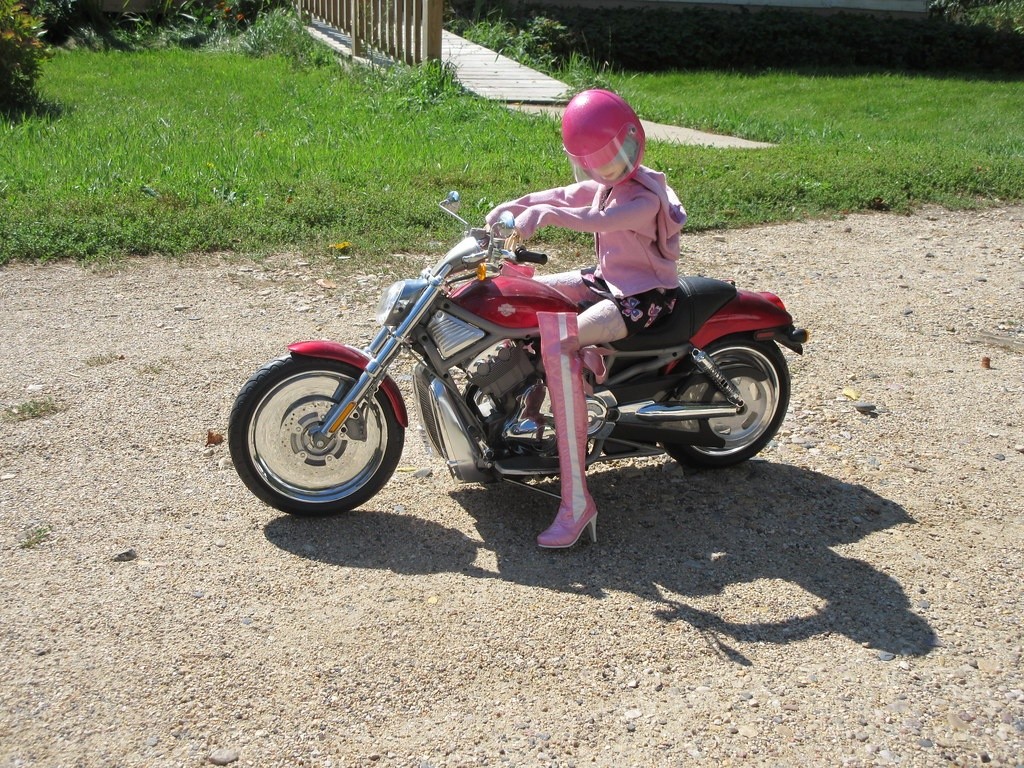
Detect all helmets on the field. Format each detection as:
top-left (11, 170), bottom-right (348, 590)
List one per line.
top-left (560, 90), bottom-right (645, 188)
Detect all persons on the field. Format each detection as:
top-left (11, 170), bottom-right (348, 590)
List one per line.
top-left (483, 89), bottom-right (683, 549)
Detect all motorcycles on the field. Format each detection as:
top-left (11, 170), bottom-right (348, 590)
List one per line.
top-left (228, 190), bottom-right (810, 518)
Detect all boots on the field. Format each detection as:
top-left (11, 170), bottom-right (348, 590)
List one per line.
top-left (536, 312), bottom-right (600, 548)
